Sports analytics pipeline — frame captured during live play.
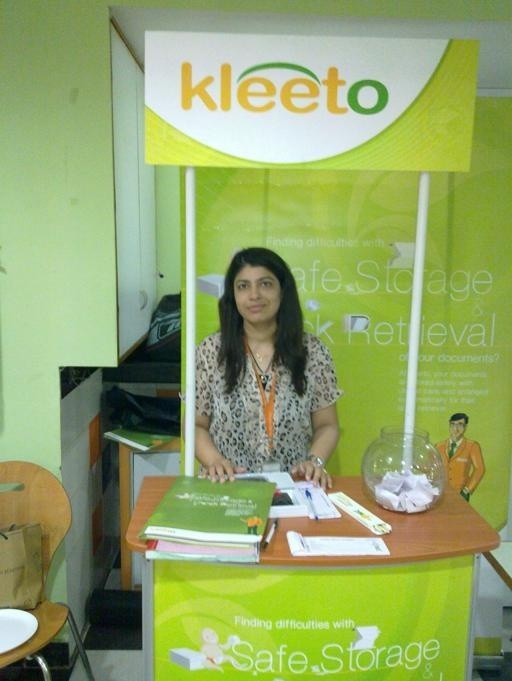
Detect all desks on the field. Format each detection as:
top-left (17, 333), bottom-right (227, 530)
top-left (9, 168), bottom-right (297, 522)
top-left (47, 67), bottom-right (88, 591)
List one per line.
top-left (125, 474), bottom-right (501, 680)
top-left (118, 425), bottom-right (181, 590)
top-left (482, 537), bottom-right (511, 593)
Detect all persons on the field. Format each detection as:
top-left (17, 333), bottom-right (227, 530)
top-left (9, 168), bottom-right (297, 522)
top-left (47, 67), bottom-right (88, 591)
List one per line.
top-left (430, 412), bottom-right (486, 503)
top-left (195, 247), bottom-right (341, 495)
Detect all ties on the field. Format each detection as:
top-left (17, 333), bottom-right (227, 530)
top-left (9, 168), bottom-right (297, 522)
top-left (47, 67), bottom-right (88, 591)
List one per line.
top-left (449, 442), bottom-right (456, 459)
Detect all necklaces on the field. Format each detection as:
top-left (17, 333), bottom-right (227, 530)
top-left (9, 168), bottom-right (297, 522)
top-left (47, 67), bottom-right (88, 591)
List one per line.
top-left (245, 335), bottom-right (280, 387)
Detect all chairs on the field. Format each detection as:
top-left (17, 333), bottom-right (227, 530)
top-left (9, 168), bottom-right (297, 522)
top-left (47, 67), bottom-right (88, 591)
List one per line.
top-left (0, 461), bottom-right (94, 680)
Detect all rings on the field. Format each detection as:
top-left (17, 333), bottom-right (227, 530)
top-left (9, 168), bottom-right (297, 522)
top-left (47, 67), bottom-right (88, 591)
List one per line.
top-left (322, 468), bottom-right (327, 474)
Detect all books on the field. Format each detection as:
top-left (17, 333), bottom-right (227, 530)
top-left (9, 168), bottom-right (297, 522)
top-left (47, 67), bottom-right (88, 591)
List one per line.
top-left (102, 426), bottom-right (176, 454)
top-left (137, 478), bottom-right (276, 565)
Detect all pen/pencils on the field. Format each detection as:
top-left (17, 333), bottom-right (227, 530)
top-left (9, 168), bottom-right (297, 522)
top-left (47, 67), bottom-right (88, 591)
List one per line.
top-left (263, 518), bottom-right (278, 552)
top-left (305, 488), bottom-right (318, 520)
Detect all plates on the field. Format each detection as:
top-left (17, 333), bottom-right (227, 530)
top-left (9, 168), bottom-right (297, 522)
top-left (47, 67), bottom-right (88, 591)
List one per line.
top-left (1, 609), bottom-right (39, 657)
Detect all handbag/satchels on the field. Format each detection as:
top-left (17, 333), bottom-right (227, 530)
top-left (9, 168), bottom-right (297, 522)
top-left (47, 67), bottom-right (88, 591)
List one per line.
top-left (0, 523), bottom-right (42, 611)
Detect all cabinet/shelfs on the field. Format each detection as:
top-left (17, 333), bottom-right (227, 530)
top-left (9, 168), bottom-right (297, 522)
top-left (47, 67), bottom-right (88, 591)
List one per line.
top-left (0, 14), bottom-right (156, 368)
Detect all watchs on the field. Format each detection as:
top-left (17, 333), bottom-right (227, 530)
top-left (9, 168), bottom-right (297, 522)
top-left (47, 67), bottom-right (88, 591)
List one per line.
top-left (305, 453), bottom-right (327, 469)
